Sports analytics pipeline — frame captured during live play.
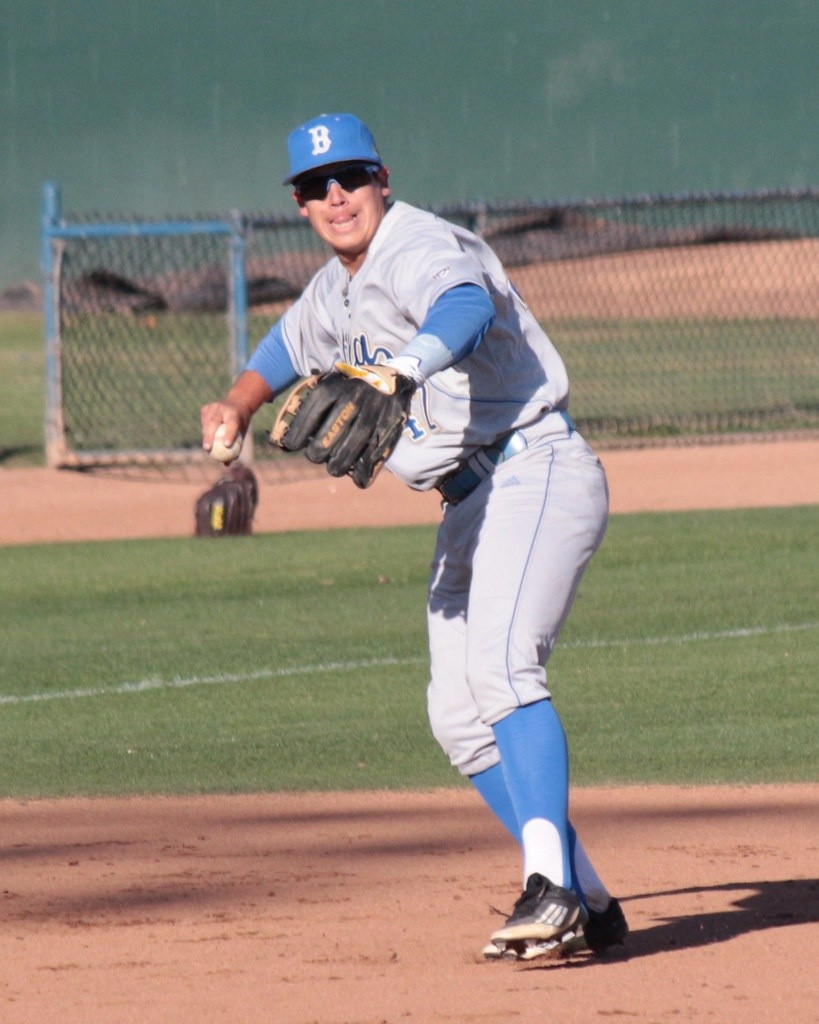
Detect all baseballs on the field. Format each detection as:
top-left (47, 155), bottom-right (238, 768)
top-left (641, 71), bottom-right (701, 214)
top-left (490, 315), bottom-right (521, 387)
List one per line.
top-left (210, 423), bottom-right (244, 462)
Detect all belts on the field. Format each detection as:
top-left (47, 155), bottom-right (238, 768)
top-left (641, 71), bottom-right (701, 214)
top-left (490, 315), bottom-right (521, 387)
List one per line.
top-left (436, 407), bottom-right (576, 507)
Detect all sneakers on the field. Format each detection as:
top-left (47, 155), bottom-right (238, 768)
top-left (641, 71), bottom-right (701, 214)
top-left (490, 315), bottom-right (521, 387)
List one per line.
top-left (541, 897), bottom-right (630, 957)
top-left (481, 873), bottom-right (593, 962)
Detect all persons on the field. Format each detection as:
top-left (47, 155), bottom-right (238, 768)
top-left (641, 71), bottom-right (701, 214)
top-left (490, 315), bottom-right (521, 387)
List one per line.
top-left (201, 112), bottom-right (628, 973)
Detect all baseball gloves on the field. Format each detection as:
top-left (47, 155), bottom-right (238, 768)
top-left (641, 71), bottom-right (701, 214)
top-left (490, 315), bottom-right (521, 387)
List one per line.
top-left (267, 363), bottom-right (425, 490)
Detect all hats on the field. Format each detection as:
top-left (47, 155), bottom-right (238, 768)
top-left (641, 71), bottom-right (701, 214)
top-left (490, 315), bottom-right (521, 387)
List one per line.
top-left (281, 112), bottom-right (382, 186)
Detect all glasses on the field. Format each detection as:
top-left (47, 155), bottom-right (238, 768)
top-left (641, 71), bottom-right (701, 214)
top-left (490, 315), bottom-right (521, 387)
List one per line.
top-left (298, 167), bottom-right (372, 200)
